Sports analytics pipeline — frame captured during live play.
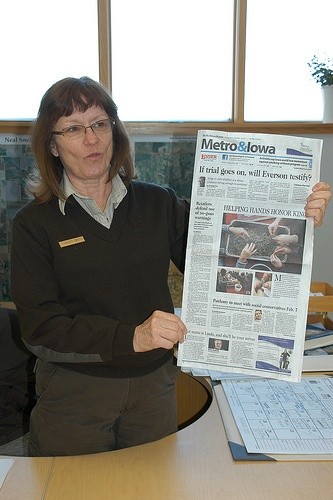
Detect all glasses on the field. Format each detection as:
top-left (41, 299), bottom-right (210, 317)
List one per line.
top-left (51, 119), bottom-right (115, 141)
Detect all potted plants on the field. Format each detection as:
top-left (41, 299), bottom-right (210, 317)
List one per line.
top-left (308, 53), bottom-right (333, 123)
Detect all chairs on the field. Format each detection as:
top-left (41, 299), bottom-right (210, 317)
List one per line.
top-left (0, 304), bottom-right (39, 458)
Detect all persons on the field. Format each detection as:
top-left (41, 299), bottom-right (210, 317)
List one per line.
top-left (213, 339), bottom-right (222, 350)
top-left (255, 310), bottom-right (262, 320)
top-left (9, 76), bottom-right (333, 457)
top-left (222, 216), bottom-right (304, 272)
top-left (216, 268), bottom-right (272, 297)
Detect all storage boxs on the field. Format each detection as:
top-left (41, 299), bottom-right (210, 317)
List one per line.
top-left (308, 281), bottom-right (333, 312)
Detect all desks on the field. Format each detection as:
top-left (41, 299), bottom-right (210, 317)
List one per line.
top-left (0, 312), bottom-right (333, 500)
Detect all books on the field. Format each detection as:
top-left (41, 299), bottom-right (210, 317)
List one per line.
top-left (301, 323), bottom-right (333, 372)
